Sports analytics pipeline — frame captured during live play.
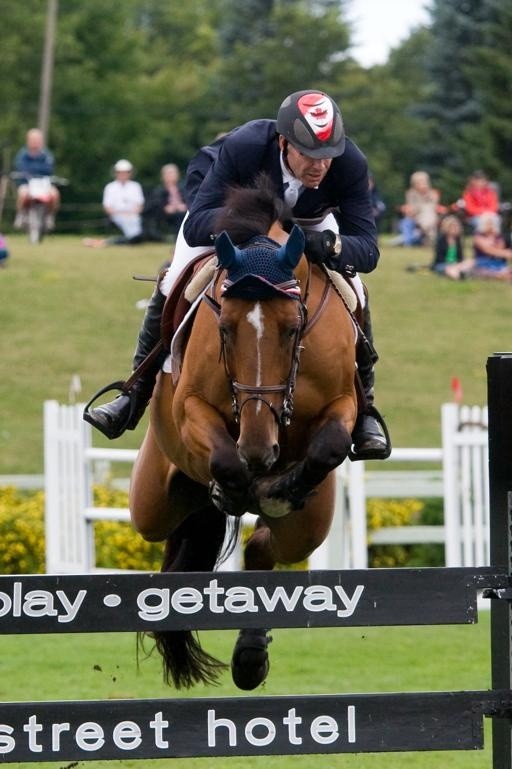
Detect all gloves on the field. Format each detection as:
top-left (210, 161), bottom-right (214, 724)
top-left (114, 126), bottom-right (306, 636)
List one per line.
top-left (302, 230), bottom-right (336, 264)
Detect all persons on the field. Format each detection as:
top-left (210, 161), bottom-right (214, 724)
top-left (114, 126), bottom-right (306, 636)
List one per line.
top-left (7, 124), bottom-right (62, 231)
top-left (139, 161), bottom-right (189, 232)
top-left (463, 209), bottom-right (512, 282)
top-left (367, 176), bottom-right (384, 221)
top-left (74, 88), bottom-right (395, 464)
top-left (428, 215), bottom-right (473, 280)
top-left (102, 155), bottom-right (149, 239)
top-left (397, 169), bottom-right (442, 247)
top-left (458, 170), bottom-right (501, 213)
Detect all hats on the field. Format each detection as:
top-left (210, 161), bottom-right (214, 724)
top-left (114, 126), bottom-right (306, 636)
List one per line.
top-left (276, 90), bottom-right (346, 160)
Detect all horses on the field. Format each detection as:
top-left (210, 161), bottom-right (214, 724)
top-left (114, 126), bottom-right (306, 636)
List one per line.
top-left (127, 170), bottom-right (361, 693)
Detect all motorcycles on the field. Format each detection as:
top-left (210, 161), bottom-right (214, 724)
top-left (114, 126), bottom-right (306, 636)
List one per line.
top-left (6, 170), bottom-right (71, 245)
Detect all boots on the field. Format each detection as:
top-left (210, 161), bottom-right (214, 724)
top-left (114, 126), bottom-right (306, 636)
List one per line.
top-left (92, 270), bottom-right (166, 430)
top-left (353, 282), bottom-right (387, 452)
